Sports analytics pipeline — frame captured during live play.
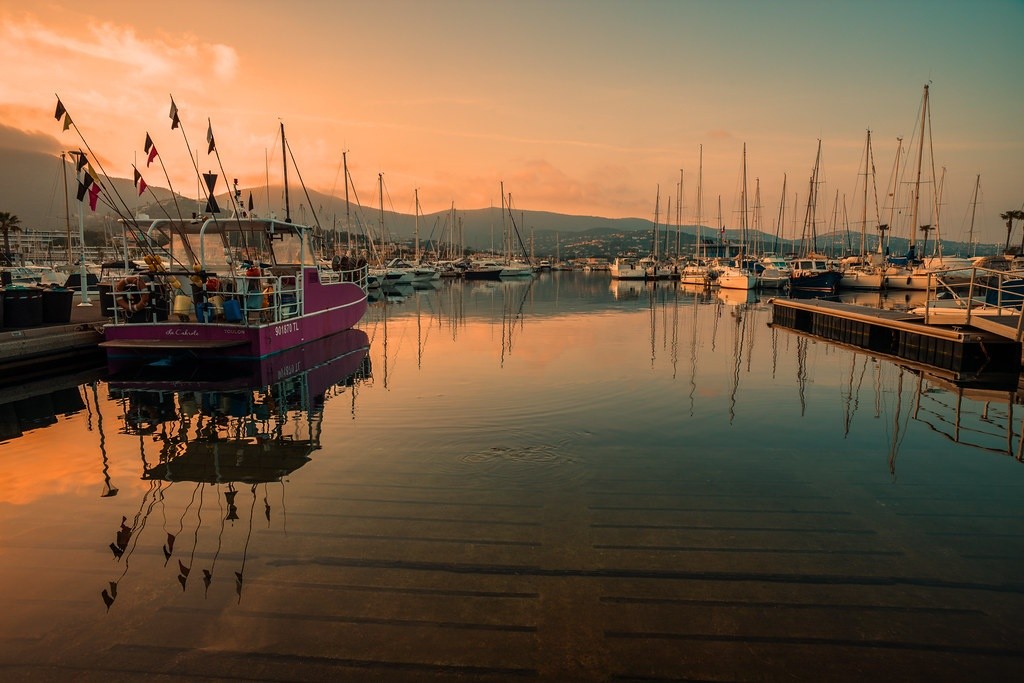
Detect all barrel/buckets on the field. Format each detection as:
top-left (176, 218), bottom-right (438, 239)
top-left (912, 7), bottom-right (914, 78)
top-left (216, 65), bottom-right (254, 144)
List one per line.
top-left (223, 299), bottom-right (242, 322)
top-left (207, 295), bottom-right (224, 315)
top-left (173, 296), bottom-right (192, 316)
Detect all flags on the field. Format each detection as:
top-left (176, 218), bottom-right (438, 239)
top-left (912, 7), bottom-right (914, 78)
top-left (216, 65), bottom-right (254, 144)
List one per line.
top-left (721, 225), bottom-right (725, 233)
top-left (54, 99), bottom-right (255, 213)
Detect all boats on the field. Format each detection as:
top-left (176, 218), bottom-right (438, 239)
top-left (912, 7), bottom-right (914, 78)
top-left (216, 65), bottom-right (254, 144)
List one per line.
top-left (86, 327), bottom-right (370, 618)
top-left (43, 87), bottom-right (371, 361)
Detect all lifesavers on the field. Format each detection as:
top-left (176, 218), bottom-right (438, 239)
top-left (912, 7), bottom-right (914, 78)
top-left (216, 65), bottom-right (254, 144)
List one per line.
top-left (114, 276), bottom-right (150, 312)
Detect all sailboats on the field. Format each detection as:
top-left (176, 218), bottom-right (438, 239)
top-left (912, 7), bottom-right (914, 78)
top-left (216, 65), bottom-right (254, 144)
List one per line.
top-left (0, 71), bottom-right (1024, 310)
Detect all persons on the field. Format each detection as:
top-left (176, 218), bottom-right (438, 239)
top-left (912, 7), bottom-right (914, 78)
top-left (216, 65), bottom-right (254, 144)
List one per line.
top-left (190, 263), bottom-right (205, 323)
